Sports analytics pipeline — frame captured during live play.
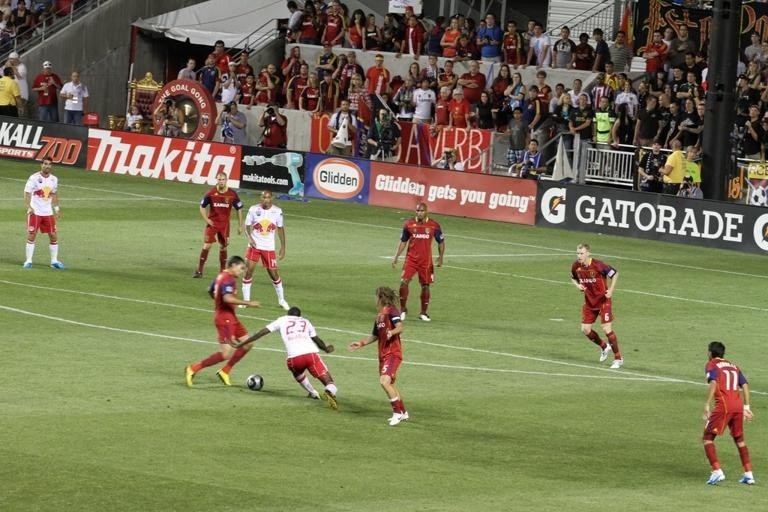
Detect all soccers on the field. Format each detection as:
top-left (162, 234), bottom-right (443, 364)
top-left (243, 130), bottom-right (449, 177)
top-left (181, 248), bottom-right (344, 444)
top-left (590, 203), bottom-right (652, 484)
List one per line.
top-left (247, 375), bottom-right (264, 391)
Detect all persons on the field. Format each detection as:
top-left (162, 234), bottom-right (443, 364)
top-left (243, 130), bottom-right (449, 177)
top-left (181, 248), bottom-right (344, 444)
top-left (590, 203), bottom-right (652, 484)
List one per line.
top-left (703, 341), bottom-right (756, 485)
top-left (121, 1), bottom-right (768, 201)
top-left (191, 173), bottom-right (242, 279)
top-left (238, 189), bottom-right (290, 310)
top-left (21, 156), bottom-right (66, 271)
top-left (569, 243), bottom-right (624, 368)
top-left (0, 1), bottom-right (105, 128)
top-left (391, 203), bottom-right (445, 323)
top-left (230, 306), bottom-right (339, 410)
top-left (347, 287), bottom-right (409, 426)
top-left (184, 256), bottom-right (253, 388)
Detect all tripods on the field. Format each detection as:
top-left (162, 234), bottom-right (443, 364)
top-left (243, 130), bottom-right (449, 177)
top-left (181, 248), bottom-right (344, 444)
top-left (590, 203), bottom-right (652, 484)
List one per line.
top-left (373, 148), bottom-right (392, 162)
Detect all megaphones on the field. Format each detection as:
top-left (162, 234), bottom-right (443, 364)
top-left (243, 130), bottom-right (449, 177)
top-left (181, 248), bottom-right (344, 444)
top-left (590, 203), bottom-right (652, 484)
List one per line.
top-left (332, 115), bottom-right (349, 148)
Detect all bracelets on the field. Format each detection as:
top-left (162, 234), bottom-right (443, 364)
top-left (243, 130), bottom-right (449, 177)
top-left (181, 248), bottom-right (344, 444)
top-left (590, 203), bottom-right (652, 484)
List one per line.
top-left (360, 342), bottom-right (365, 346)
top-left (395, 254), bottom-right (399, 260)
top-left (744, 405), bottom-right (750, 411)
top-left (54, 205), bottom-right (60, 212)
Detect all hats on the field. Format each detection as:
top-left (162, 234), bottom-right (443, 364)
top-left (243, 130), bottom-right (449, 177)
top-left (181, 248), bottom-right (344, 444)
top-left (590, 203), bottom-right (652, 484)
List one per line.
top-left (9, 52), bottom-right (19, 59)
top-left (43, 61), bottom-right (52, 68)
top-left (684, 176), bottom-right (693, 182)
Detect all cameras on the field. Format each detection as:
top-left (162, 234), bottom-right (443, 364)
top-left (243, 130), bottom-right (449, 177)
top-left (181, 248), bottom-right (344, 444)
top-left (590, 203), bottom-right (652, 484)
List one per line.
top-left (224, 104), bottom-right (231, 113)
top-left (446, 151), bottom-right (452, 160)
top-left (268, 108), bottom-right (274, 116)
top-left (165, 100), bottom-right (172, 106)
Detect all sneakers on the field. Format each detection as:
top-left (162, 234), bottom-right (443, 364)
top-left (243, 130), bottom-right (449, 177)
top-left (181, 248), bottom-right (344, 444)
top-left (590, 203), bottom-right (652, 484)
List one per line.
top-left (390, 411), bottom-right (404, 425)
top-left (24, 261), bottom-right (33, 269)
top-left (216, 369), bottom-right (232, 387)
top-left (739, 471), bottom-right (755, 485)
top-left (324, 390), bottom-right (337, 410)
top-left (400, 310), bottom-right (407, 321)
top-left (308, 393), bottom-right (321, 399)
top-left (419, 314), bottom-right (431, 321)
top-left (610, 357), bottom-right (623, 369)
top-left (186, 366), bottom-right (195, 387)
top-left (278, 300), bottom-right (290, 310)
top-left (707, 468), bottom-right (725, 485)
top-left (388, 411), bottom-right (409, 421)
top-left (192, 272), bottom-right (202, 278)
top-left (600, 344), bottom-right (611, 362)
top-left (50, 261), bottom-right (63, 270)
